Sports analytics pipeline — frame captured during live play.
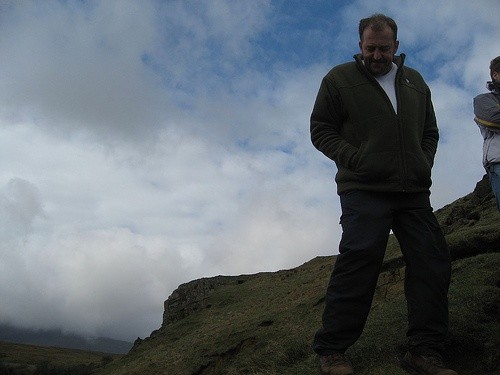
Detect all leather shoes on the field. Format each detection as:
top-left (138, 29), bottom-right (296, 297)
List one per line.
top-left (317, 353), bottom-right (353, 375)
top-left (402, 351), bottom-right (458, 374)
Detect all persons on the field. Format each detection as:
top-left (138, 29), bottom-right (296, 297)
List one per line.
top-left (309, 14), bottom-right (459, 375)
top-left (473, 57), bottom-right (500, 212)
